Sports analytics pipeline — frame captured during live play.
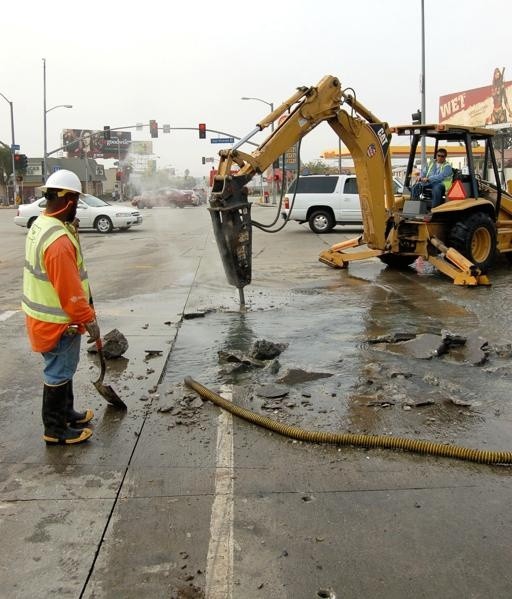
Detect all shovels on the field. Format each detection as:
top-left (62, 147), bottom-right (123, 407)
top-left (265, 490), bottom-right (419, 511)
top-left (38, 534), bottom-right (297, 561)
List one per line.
top-left (75, 222), bottom-right (128, 409)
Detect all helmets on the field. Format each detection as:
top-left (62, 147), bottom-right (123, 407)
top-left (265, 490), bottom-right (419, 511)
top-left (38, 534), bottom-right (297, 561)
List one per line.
top-left (40, 169), bottom-right (86, 198)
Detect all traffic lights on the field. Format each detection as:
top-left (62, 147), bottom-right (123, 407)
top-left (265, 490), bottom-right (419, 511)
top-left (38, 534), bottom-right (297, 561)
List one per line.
top-left (409, 109), bottom-right (422, 141)
top-left (20, 154), bottom-right (28, 169)
top-left (16, 176), bottom-right (23, 181)
top-left (274, 174), bottom-right (280, 182)
top-left (198, 124), bottom-right (207, 139)
top-left (14, 154), bottom-right (21, 169)
top-left (150, 121), bottom-right (159, 139)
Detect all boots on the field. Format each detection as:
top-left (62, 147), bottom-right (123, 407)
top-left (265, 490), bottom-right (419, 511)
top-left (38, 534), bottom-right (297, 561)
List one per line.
top-left (65, 378), bottom-right (94, 426)
top-left (41, 379), bottom-right (93, 444)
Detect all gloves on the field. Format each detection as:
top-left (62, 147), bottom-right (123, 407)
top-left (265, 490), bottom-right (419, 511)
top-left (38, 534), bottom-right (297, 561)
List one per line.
top-left (84, 317), bottom-right (101, 344)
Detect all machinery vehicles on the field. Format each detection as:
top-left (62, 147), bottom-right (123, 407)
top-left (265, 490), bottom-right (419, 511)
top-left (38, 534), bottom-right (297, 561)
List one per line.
top-left (202, 72), bottom-right (512, 308)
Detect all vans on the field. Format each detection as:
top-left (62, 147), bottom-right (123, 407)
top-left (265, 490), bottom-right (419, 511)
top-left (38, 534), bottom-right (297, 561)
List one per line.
top-left (280, 171), bottom-right (411, 236)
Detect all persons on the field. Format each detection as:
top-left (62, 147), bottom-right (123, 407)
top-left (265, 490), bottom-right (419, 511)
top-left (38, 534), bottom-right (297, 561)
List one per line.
top-left (411, 149), bottom-right (453, 207)
top-left (491, 68), bottom-right (512, 124)
top-left (21, 169), bottom-right (100, 444)
top-left (79, 130), bottom-right (94, 158)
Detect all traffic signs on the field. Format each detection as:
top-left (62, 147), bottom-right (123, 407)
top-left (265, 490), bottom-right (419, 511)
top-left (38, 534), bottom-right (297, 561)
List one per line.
top-left (10, 144), bottom-right (20, 150)
top-left (210, 137), bottom-right (235, 143)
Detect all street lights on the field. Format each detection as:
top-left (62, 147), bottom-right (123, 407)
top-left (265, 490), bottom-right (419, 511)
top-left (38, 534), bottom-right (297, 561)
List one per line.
top-left (41, 103), bottom-right (73, 184)
top-left (116, 135), bottom-right (134, 199)
top-left (239, 96), bottom-right (278, 204)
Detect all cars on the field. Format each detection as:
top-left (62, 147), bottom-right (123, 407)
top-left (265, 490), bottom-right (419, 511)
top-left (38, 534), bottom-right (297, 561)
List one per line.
top-left (12, 191), bottom-right (145, 234)
top-left (131, 186), bottom-right (208, 209)
